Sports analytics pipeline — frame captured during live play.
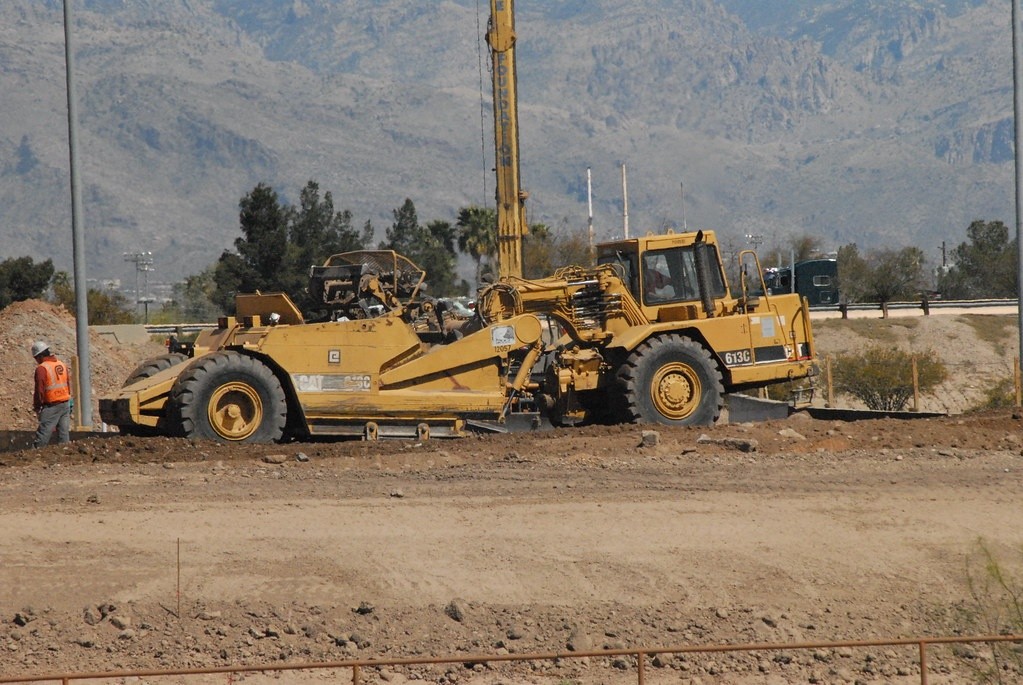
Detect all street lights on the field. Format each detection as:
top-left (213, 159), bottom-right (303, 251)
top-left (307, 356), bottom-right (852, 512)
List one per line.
top-left (139, 298), bottom-right (155, 322)
top-left (938, 248), bottom-right (946, 268)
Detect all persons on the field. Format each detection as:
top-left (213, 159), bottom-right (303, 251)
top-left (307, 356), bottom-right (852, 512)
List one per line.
top-left (31, 342), bottom-right (70, 450)
top-left (636, 258), bottom-right (672, 303)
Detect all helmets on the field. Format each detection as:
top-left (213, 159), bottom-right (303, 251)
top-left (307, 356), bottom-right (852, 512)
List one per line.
top-left (31, 342), bottom-right (49, 358)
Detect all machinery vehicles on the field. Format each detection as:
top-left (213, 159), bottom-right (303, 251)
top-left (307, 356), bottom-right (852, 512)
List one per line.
top-left (97, 0), bottom-right (816, 449)
